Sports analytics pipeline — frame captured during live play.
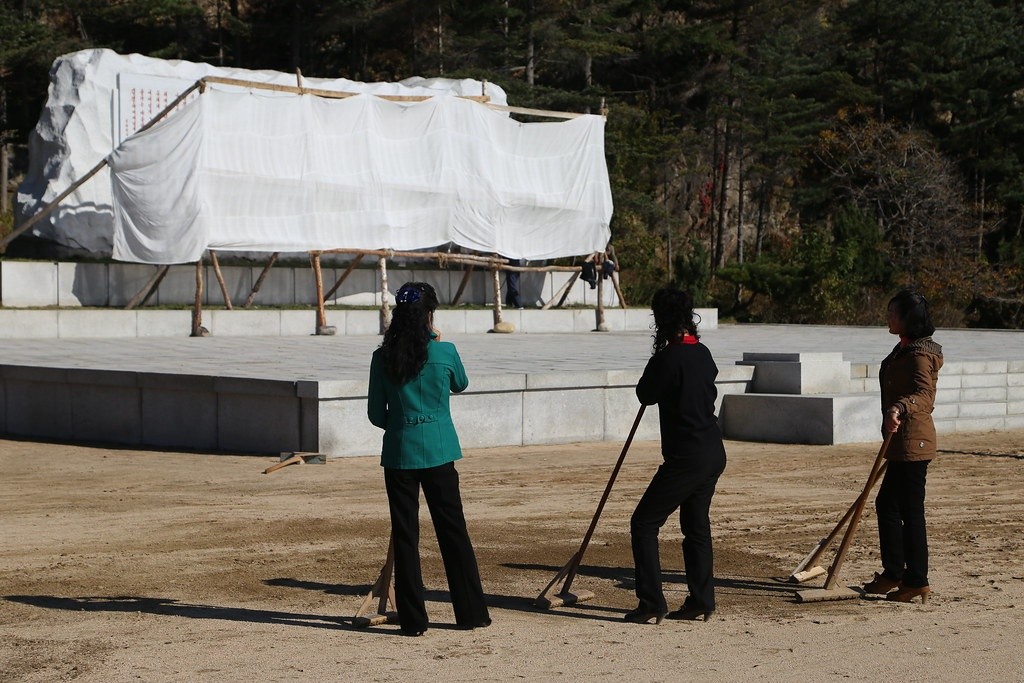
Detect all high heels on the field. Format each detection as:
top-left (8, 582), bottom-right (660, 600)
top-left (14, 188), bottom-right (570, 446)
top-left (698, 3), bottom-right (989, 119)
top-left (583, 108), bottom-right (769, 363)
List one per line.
top-left (864, 569), bottom-right (905, 594)
top-left (669, 596), bottom-right (714, 622)
top-left (887, 583), bottom-right (929, 604)
top-left (625, 608), bottom-right (667, 624)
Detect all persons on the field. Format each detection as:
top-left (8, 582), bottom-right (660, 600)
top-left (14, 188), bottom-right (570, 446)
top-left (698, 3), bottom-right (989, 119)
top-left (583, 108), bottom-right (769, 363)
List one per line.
top-left (864, 291), bottom-right (943, 604)
top-left (503, 257), bottom-right (524, 310)
top-left (624, 287), bottom-right (727, 624)
top-left (367, 282), bottom-right (491, 637)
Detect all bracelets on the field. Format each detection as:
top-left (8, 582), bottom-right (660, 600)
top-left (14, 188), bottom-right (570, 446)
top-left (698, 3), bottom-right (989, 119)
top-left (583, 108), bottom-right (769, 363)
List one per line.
top-left (887, 410), bottom-right (900, 415)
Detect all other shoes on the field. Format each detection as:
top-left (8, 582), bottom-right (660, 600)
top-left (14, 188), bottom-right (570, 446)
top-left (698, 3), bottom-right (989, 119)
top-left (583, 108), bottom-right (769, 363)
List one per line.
top-left (465, 617), bottom-right (491, 630)
top-left (401, 627), bottom-right (426, 636)
